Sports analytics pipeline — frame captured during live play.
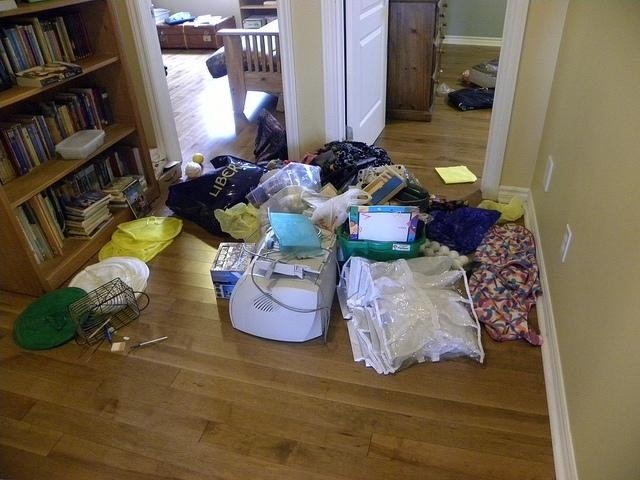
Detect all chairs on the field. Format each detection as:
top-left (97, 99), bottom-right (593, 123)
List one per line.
top-left (216, 18), bottom-right (284, 114)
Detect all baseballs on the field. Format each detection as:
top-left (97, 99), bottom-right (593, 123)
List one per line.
top-left (420, 239), bottom-right (468, 270)
top-left (193, 153), bottom-right (204, 164)
top-left (185, 162), bottom-right (202, 177)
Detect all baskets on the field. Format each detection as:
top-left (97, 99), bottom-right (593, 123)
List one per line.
top-left (68, 277), bottom-right (149, 347)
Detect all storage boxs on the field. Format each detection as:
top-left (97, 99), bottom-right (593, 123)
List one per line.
top-left (156, 17), bottom-right (190, 49)
top-left (54, 129), bottom-right (106, 162)
top-left (210, 242), bottom-right (256, 300)
top-left (337, 224), bottom-right (426, 262)
top-left (185, 15), bottom-right (236, 49)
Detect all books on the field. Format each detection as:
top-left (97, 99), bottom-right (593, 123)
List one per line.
top-left (0, 9), bottom-right (151, 265)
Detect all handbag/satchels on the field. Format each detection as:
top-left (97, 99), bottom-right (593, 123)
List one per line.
top-left (254, 108), bottom-right (288, 162)
top-left (425, 200), bottom-right (501, 255)
top-left (165, 155), bottom-right (268, 237)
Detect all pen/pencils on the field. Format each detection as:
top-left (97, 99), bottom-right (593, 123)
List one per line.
top-left (89, 315), bottom-right (113, 343)
top-left (130, 336), bottom-right (169, 349)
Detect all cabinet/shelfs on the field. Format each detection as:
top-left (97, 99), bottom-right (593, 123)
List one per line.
top-left (0, 0), bottom-right (160, 297)
top-left (239, 0), bottom-right (277, 29)
top-left (386, 0), bottom-right (449, 121)
